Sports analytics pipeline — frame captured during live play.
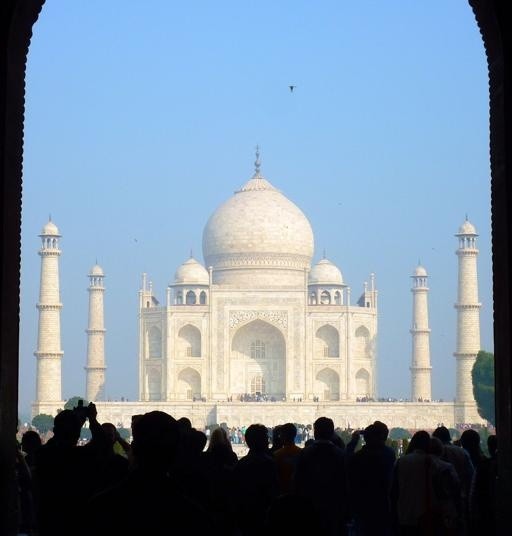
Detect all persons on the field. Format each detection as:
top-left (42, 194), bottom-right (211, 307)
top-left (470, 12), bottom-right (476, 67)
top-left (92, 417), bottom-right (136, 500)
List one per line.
top-left (14, 392), bottom-right (499, 535)
